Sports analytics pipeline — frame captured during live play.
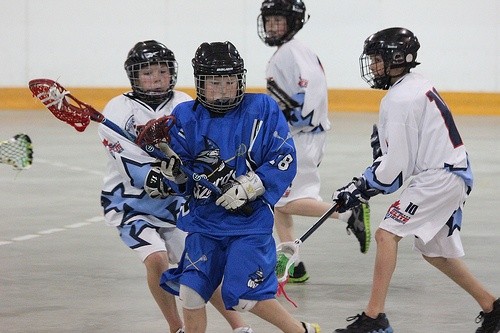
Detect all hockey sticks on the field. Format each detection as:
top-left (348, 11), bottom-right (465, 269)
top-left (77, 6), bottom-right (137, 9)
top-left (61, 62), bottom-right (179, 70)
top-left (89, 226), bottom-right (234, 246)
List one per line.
top-left (276, 204), bottom-right (339, 297)
top-left (136, 115), bottom-right (177, 147)
top-left (0, 134), bottom-right (33, 170)
top-left (29, 79), bottom-right (255, 217)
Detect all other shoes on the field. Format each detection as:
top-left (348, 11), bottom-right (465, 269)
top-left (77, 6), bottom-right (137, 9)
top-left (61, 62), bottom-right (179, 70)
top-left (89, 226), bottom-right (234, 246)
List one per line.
top-left (301, 322), bottom-right (320, 333)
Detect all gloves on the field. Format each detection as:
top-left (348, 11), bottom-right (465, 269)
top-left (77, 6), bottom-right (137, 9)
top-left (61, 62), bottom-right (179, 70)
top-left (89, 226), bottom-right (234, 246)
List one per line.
top-left (161, 156), bottom-right (189, 184)
top-left (369, 122), bottom-right (384, 161)
top-left (215, 170), bottom-right (266, 210)
top-left (332, 175), bottom-right (381, 213)
top-left (144, 167), bottom-right (176, 199)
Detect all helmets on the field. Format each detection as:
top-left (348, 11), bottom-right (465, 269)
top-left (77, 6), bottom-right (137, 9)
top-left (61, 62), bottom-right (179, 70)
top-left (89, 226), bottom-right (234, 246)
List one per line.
top-left (359, 27), bottom-right (422, 91)
top-left (191, 41), bottom-right (248, 113)
top-left (125, 40), bottom-right (178, 105)
top-left (257, 0), bottom-right (306, 46)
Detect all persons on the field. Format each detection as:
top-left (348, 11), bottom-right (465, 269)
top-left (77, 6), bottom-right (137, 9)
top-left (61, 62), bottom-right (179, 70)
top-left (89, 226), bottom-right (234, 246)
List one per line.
top-left (256, 0), bottom-right (372, 283)
top-left (150, 41), bottom-right (320, 333)
top-left (331, 27), bottom-right (500, 333)
top-left (97, 40), bottom-right (252, 333)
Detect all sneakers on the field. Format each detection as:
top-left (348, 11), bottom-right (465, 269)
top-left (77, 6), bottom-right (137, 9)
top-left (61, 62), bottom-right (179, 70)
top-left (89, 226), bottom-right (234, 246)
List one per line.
top-left (475, 297), bottom-right (500, 333)
top-left (274, 256), bottom-right (309, 283)
top-left (345, 201), bottom-right (371, 254)
top-left (335, 312), bottom-right (394, 333)
